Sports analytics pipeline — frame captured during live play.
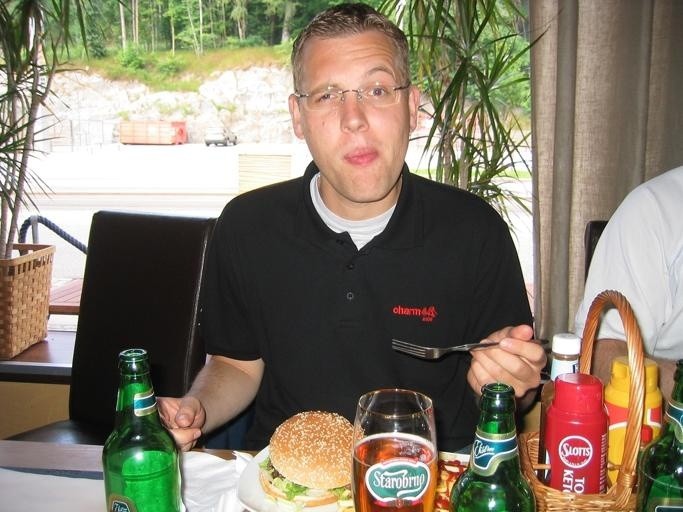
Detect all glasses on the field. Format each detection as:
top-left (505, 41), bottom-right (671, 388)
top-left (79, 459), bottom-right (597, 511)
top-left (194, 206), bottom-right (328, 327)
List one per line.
top-left (293, 81), bottom-right (411, 109)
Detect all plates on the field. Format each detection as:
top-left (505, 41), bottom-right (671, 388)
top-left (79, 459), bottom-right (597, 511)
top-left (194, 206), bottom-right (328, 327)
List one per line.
top-left (233, 442), bottom-right (351, 511)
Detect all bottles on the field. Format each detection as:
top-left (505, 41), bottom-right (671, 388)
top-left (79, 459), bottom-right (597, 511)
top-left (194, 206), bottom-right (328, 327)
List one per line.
top-left (100, 347), bottom-right (183, 512)
top-left (533, 331), bottom-right (683, 512)
top-left (445, 380), bottom-right (538, 511)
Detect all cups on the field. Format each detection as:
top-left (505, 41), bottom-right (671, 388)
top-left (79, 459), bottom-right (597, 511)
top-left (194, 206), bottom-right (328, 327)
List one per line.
top-left (350, 387), bottom-right (438, 511)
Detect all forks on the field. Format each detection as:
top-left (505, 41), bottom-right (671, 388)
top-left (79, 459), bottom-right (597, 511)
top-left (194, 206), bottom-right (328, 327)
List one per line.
top-left (389, 335), bottom-right (499, 361)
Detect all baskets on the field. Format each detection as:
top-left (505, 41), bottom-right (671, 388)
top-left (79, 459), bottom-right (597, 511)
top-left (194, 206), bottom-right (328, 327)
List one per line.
top-left (518, 288), bottom-right (646, 512)
top-left (0, 240), bottom-right (56, 361)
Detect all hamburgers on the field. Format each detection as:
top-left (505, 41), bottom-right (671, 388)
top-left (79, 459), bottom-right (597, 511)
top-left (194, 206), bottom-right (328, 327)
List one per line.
top-left (259, 410), bottom-right (363, 508)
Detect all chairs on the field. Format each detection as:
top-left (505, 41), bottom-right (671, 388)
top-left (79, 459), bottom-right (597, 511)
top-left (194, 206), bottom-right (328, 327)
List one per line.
top-left (0, 211), bottom-right (205, 444)
top-left (183, 219), bottom-right (254, 452)
top-left (16, 215), bottom-right (88, 322)
top-left (585, 221), bottom-right (610, 285)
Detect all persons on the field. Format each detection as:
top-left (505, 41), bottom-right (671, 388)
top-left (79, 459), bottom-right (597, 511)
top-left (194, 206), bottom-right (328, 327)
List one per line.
top-left (574, 165), bottom-right (683, 413)
top-left (155, 2), bottom-right (545, 452)
